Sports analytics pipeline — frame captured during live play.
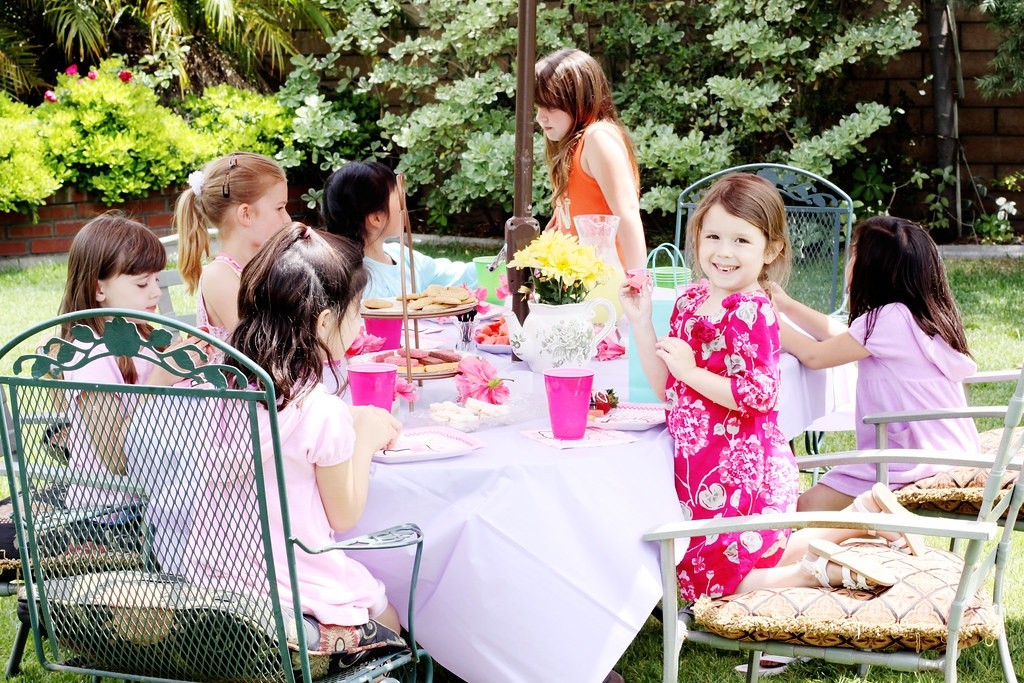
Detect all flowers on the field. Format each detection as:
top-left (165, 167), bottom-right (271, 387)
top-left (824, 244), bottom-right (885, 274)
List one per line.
top-left (509, 232), bottom-right (608, 304)
top-left (323, 333), bottom-right (418, 399)
top-left (450, 287), bottom-right (487, 324)
top-left (455, 356), bottom-right (513, 406)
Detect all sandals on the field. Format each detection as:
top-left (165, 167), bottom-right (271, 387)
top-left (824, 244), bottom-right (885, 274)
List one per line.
top-left (802, 542), bottom-right (895, 594)
top-left (853, 486), bottom-right (923, 558)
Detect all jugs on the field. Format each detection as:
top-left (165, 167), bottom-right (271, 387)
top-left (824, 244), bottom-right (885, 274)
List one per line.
top-left (573, 215), bottom-right (627, 326)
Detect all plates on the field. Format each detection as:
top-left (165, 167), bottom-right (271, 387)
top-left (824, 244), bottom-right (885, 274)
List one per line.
top-left (370, 426), bottom-right (485, 464)
top-left (475, 342), bottom-right (512, 353)
top-left (587, 403), bottom-right (666, 430)
top-left (426, 300), bottom-right (510, 323)
top-left (648, 266), bottom-right (691, 289)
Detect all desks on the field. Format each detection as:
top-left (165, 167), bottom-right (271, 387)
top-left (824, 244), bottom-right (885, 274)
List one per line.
top-left (358, 409), bottom-right (693, 683)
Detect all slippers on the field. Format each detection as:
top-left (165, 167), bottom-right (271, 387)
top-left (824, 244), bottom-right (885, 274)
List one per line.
top-left (735, 648), bottom-right (811, 676)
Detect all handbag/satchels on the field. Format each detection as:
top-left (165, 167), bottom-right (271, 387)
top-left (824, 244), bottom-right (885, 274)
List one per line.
top-left (628, 243), bottom-right (687, 403)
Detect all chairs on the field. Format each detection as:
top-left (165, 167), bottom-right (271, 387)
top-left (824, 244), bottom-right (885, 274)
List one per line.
top-left (0, 161), bottom-right (1022, 683)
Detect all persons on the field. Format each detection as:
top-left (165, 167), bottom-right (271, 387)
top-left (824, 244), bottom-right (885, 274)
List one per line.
top-left (323, 160), bottom-right (475, 299)
top-left (176, 152), bottom-right (292, 343)
top-left (57, 210), bottom-right (218, 525)
top-left (129, 222), bottom-right (401, 636)
top-left (620, 172), bottom-right (924, 604)
top-left (770, 217), bottom-right (982, 511)
top-left (535, 48), bottom-right (647, 272)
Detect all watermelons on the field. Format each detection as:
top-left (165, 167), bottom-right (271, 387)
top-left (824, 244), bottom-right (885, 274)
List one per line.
top-left (474, 316), bottom-right (511, 345)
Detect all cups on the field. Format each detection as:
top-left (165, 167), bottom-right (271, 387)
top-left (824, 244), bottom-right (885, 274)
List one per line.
top-left (187, 326), bottom-right (211, 349)
top-left (543, 367), bottom-right (596, 440)
top-left (472, 256), bottom-right (507, 305)
top-left (628, 268), bottom-right (651, 296)
top-left (364, 318), bottom-right (403, 351)
top-left (346, 363), bottom-right (398, 415)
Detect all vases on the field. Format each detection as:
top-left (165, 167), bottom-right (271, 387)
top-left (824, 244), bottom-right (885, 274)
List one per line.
top-left (453, 323), bottom-right (478, 353)
top-left (503, 299), bottom-right (616, 374)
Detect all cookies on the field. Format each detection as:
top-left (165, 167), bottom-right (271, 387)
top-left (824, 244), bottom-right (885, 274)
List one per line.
top-left (370, 345), bottom-right (464, 375)
top-left (363, 283), bottom-right (475, 312)
